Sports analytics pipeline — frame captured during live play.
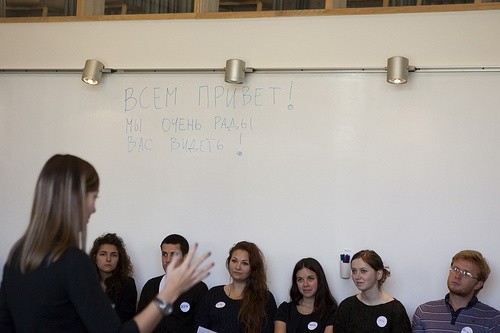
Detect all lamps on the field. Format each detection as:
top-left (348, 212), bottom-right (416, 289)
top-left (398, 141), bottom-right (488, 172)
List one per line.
top-left (82, 60), bottom-right (111, 85)
top-left (385, 56), bottom-right (415, 84)
top-left (225, 59), bottom-right (253, 84)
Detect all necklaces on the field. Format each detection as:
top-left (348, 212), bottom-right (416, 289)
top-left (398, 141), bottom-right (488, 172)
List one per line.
top-left (229, 285), bottom-right (242, 298)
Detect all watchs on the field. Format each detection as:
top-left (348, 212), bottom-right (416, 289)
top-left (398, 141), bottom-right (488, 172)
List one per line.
top-left (153, 297), bottom-right (174, 318)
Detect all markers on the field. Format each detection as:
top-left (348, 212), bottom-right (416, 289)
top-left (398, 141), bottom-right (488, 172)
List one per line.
top-left (340, 253), bottom-right (351, 263)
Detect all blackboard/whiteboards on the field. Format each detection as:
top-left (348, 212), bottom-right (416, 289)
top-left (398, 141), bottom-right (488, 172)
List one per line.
top-left (1, 71), bottom-right (499, 324)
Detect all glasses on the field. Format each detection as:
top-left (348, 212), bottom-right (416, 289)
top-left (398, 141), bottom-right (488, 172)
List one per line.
top-left (449, 265), bottom-right (479, 281)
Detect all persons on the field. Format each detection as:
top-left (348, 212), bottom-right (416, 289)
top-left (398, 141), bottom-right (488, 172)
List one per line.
top-left (0, 154), bottom-right (216, 333)
top-left (137, 234), bottom-right (209, 333)
top-left (333, 250), bottom-right (412, 333)
top-left (89, 233), bottom-right (138, 323)
top-left (274, 257), bottom-right (338, 333)
top-left (412, 250), bottom-right (500, 333)
top-left (209, 241), bottom-right (278, 333)
